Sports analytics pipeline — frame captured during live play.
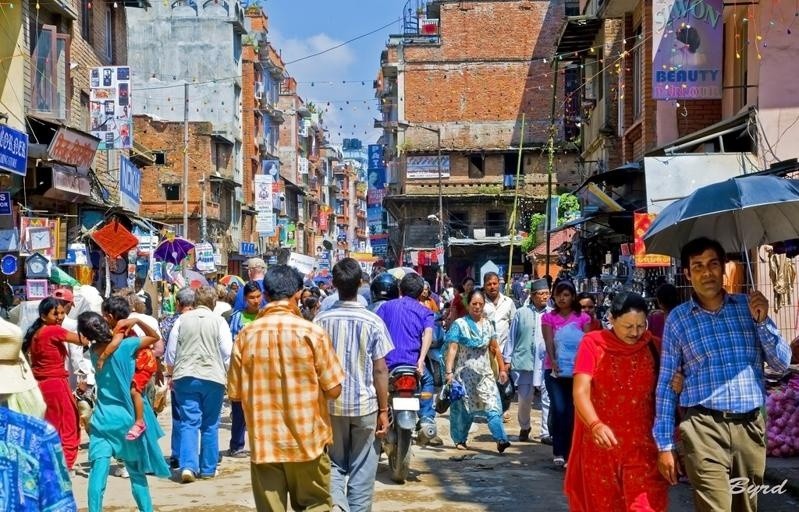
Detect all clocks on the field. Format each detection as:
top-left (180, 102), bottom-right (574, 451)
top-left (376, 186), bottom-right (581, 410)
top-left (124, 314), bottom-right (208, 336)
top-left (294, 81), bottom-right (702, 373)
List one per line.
top-left (0, 255), bottom-right (18, 275)
top-left (0, 226), bottom-right (21, 252)
top-left (26, 279), bottom-right (48, 300)
top-left (26, 226), bottom-right (52, 251)
top-left (25, 252), bottom-right (50, 278)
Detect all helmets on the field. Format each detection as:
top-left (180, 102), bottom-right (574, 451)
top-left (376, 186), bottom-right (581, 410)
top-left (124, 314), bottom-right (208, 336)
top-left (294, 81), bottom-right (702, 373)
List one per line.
top-left (370, 272), bottom-right (399, 302)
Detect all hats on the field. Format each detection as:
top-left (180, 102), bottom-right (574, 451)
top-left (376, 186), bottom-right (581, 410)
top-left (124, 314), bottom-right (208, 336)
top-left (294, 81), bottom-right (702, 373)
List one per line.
top-left (530, 278), bottom-right (549, 292)
top-left (247, 257), bottom-right (266, 269)
top-left (51, 288), bottom-right (76, 308)
top-left (0, 316), bottom-right (40, 395)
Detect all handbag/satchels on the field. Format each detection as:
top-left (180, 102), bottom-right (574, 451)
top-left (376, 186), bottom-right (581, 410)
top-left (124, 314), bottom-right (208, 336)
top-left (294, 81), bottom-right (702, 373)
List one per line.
top-left (435, 384), bottom-right (453, 414)
top-left (154, 381), bottom-right (168, 412)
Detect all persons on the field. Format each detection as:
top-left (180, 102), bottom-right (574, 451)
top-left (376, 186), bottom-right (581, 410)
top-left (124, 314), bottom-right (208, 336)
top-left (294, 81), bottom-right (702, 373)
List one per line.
top-left (670, 26), bottom-right (706, 66)
top-left (264, 162), bottom-right (278, 181)
top-left (0, 237), bottom-right (793, 512)
top-left (368, 171), bottom-right (379, 190)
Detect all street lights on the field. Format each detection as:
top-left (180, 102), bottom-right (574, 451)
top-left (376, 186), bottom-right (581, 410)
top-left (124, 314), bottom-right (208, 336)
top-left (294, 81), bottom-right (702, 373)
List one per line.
top-left (397, 119), bottom-right (444, 294)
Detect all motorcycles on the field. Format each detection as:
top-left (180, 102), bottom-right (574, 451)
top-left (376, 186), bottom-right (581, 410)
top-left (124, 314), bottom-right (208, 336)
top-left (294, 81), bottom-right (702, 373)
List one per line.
top-left (382, 365), bottom-right (438, 483)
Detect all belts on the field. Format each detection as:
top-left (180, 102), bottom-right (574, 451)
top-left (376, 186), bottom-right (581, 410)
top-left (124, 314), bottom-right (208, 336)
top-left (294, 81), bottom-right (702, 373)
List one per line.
top-left (695, 404), bottom-right (756, 421)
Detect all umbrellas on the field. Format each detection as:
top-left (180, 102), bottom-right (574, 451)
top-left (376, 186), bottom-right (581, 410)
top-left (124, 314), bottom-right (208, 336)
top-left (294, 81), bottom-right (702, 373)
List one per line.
top-left (641, 173), bottom-right (799, 292)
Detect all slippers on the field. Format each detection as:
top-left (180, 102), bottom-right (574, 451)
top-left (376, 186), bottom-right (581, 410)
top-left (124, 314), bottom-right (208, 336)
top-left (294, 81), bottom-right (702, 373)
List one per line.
top-left (456, 442), bottom-right (468, 450)
top-left (497, 439), bottom-right (511, 452)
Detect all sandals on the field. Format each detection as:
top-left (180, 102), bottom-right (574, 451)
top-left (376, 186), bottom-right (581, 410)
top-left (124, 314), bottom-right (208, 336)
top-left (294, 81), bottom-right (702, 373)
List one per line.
top-left (126, 420), bottom-right (146, 441)
top-left (554, 455), bottom-right (565, 467)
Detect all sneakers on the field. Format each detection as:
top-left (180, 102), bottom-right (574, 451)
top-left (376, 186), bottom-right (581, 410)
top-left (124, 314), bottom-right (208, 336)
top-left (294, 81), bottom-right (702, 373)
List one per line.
top-left (114, 466), bottom-right (130, 478)
top-left (541, 437), bottom-right (553, 445)
top-left (181, 469), bottom-right (195, 482)
top-left (519, 427), bottom-right (531, 441)
top-left (201, 470), bottom-right (218, 478)
top-left (231, 451), bottom-right (247, 457)
top-left (427, 436), bottom-right (443, 446)
